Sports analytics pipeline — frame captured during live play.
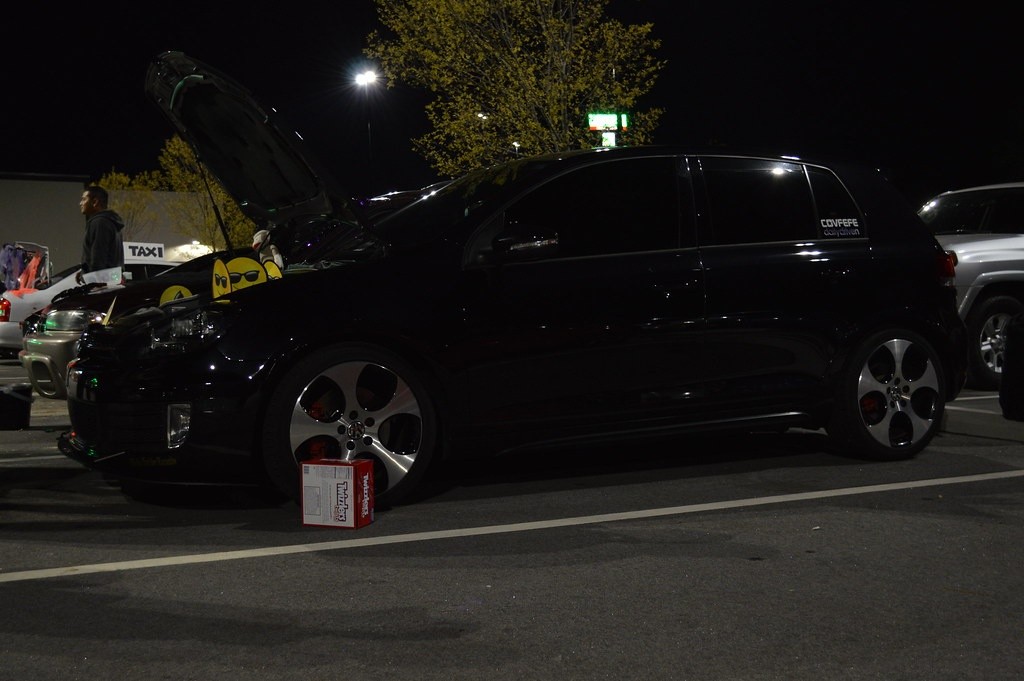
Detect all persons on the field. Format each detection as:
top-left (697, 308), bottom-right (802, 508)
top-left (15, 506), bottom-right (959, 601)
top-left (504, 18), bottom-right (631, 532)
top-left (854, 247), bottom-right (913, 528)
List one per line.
top-left (76, 186), bottom-right (125, 294)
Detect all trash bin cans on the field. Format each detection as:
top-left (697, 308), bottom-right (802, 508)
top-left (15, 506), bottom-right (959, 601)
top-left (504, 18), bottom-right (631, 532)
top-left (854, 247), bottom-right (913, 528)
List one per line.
top-left (0, 383), bottom-right (34, 430)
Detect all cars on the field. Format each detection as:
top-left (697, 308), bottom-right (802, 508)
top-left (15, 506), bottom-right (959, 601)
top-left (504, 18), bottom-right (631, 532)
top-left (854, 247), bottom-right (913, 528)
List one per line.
top-left (0, 45), bottom-right (969, 514)
top-left (916, 182), bottom-right (1024, 393)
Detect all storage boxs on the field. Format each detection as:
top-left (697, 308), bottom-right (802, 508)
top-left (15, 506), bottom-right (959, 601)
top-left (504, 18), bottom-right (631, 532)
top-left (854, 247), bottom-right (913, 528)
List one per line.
top-left (299, 456), bottom-right (376, 530)
